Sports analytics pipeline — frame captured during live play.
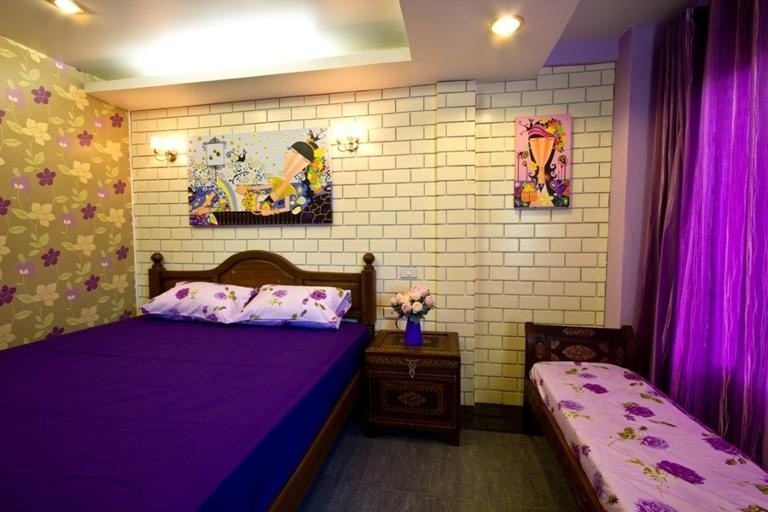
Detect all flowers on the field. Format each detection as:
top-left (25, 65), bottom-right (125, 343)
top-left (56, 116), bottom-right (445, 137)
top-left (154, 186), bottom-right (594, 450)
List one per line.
top-left (390, 286), bottom-right (435, 328)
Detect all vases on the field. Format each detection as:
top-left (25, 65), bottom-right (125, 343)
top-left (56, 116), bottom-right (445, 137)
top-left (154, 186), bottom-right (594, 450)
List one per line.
top-left (404, 318), bottom-right (422, 346)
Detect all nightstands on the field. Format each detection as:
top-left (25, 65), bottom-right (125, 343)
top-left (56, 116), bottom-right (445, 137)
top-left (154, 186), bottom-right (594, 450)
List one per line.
top-left (363, 329), bottom-right (461, 447)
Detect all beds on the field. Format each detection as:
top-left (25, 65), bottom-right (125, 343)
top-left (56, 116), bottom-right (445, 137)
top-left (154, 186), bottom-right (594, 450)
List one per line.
top-left (0, 251), bottom-right (376, 512)
top-left (521, 320), bottom-right (768, 512)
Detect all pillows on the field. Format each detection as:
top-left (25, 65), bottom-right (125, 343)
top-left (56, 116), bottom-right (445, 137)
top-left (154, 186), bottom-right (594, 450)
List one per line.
top-left (138, 280), bottom-right (257, 324)
top-left (236, 283), bottom-right (352, 329)
